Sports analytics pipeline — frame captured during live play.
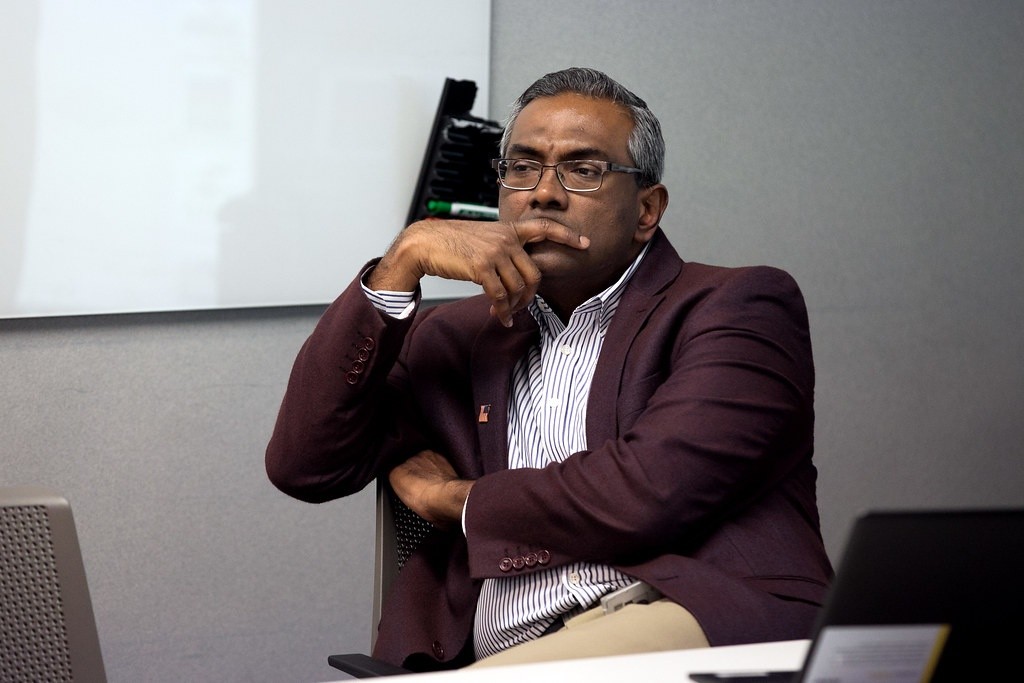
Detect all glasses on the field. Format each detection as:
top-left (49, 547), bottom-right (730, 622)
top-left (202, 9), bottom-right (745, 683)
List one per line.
top-left (491, 159), bottom-right (642, 192)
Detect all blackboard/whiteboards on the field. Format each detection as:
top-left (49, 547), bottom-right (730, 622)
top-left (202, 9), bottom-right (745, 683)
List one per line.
top-left (0, 0), bottom-right (494, 321)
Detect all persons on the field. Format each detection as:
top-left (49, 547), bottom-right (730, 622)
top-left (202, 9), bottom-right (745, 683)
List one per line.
top-left (266, 67), bottom-right (836, 672)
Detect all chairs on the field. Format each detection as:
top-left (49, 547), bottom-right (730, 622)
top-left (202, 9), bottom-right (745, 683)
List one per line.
top-left (0, 498), bottom-right (107, 683)
top-left (375, 472), bottom-right (432, 651)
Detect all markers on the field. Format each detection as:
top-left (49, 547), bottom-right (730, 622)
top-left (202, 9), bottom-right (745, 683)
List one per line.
top-left (428, 199), bottom-right (500, 220)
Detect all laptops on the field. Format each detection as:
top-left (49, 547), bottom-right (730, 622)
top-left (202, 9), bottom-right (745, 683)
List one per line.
top-left (690, 508), bottom-right (1024, 683)
